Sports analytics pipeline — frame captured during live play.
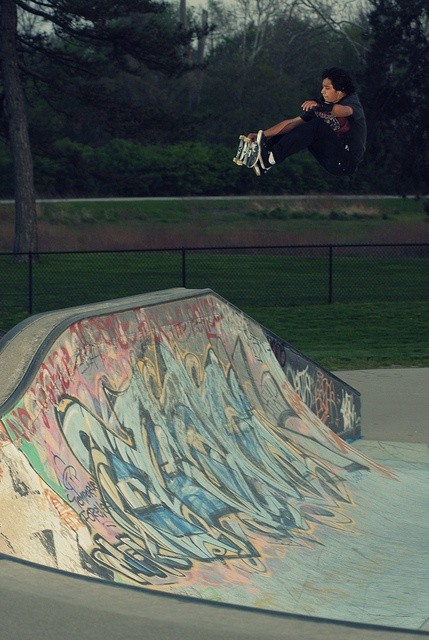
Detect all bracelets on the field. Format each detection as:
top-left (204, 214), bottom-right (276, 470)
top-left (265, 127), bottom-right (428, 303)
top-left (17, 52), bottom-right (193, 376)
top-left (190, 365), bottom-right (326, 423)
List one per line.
top-left (316, 101), bottom-right (333, 114)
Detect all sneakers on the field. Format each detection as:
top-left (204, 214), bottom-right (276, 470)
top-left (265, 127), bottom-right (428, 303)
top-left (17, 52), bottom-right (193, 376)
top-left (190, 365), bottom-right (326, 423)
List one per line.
top-left (257, 130), bottom-right (279, 169)
top-left (252, 159), bottom-right (268, 177)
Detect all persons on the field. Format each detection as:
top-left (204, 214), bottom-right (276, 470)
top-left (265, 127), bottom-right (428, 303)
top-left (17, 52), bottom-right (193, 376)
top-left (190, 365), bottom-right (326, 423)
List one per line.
top-left (248, 67), bottom-right (367, 177)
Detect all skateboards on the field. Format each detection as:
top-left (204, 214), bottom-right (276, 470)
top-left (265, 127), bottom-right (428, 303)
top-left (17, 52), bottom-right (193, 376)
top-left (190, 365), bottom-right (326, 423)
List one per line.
top-left (232, 135), bottom-right (261, 168)
